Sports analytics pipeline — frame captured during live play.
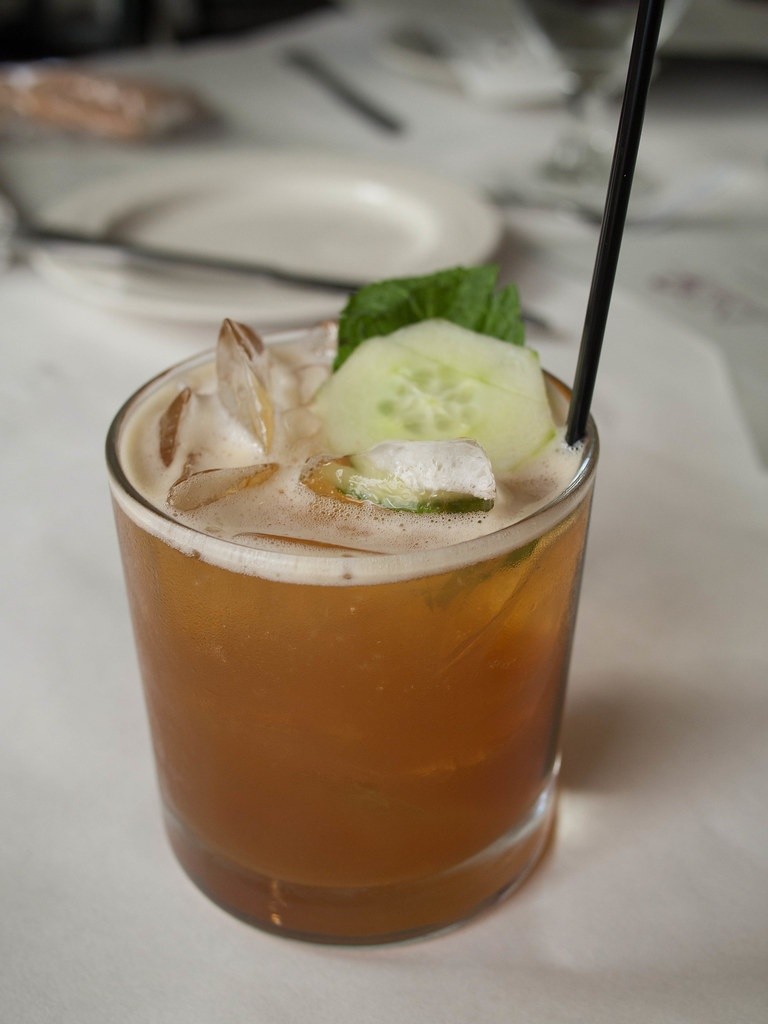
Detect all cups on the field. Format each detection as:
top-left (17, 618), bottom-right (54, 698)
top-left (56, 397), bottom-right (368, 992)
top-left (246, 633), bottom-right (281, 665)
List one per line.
top-left (104, 316), bottom-right (599, 945)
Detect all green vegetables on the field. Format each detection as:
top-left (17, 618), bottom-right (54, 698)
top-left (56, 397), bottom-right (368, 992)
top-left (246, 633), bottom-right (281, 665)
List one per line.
top-left (334, 267), bottom-right (524, 365)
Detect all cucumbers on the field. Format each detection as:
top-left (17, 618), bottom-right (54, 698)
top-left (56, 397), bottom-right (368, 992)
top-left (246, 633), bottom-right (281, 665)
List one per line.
top-left (311, 333), bottom-right (555, 475)
top-left (393, 318), bottom-right (543, 399)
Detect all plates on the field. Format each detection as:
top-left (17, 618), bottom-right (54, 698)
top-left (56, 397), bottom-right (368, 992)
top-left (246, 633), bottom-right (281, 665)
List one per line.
top-left (1, 147), bottom-right (506, 321)
